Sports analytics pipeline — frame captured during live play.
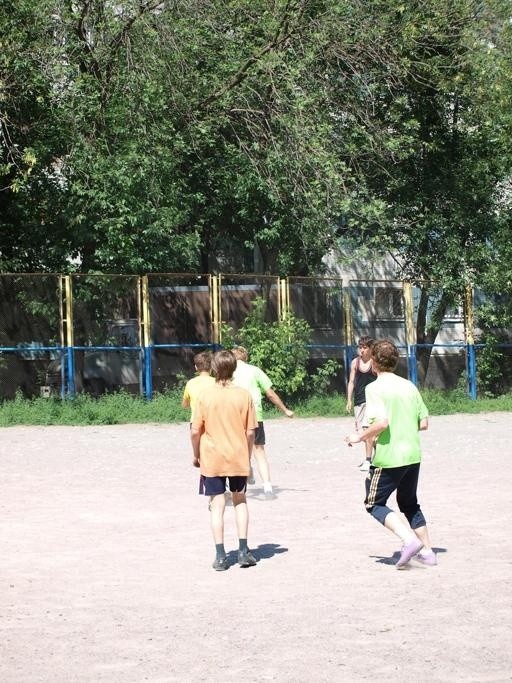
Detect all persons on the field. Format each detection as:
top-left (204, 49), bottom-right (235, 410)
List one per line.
top-left (344, 340), bottom-right (438, 570)
top-left (345, 334), bottom-right (376, 471)
top-left (189, 350), bottom-right (259, 569)
top-left (226, 345), bottom-right (294, 499)
top-left (182, 352), bottom-right (230, 509)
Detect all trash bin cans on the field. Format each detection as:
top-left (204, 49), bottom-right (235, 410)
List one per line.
top-left (443, 368), bottom-right (458, 389)
top-left (40, 385), bottom-right (51, 397)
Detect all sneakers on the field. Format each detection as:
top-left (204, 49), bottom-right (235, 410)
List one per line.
top-left (238, 551), bottom-right (257, 566)
top-left (413, 551), bottom-right (438, 566)
top-left (213, 556), bottom-right (231, 570)
top-left (395, 538), bottom-right (424, 569)
top-left (262, 483), bottom-right (273, 495)
